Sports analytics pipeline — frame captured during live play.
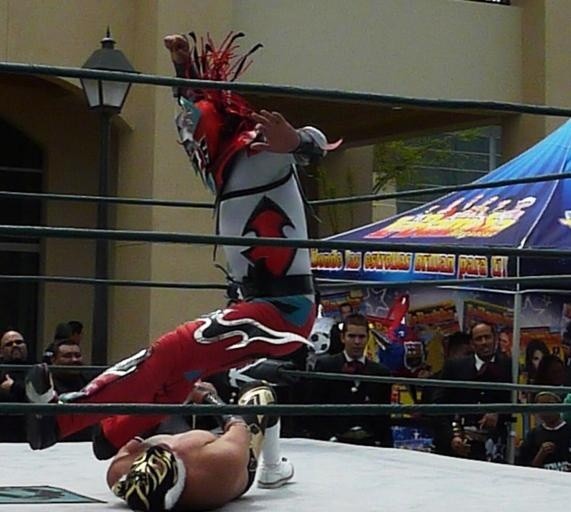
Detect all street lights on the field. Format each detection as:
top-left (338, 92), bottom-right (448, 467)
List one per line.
top-left (81, 26), bottom-right (139, 383)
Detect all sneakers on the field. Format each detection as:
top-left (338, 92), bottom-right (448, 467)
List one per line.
top-left (92, 424), bottom-right (118, 461)
top-left (23, 363), bottom-right (59, 450)
top-left (258, 457), bottom-right (294, 489)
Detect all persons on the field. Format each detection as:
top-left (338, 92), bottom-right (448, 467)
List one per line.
top-left (43, 339), bottom-right (100, 398)
top-left (311, 312), bottom-right (395, 449)
top-left (1, 329), bottom-right (38, 443)
top-left (24, 28), bottom-right (335, 461)
top-left (417, 323), bottom-right (571, 475)
top-left (329, 302), bottom-right (354, 356)
top-left (105, 380), bottom-right (296, 512)
